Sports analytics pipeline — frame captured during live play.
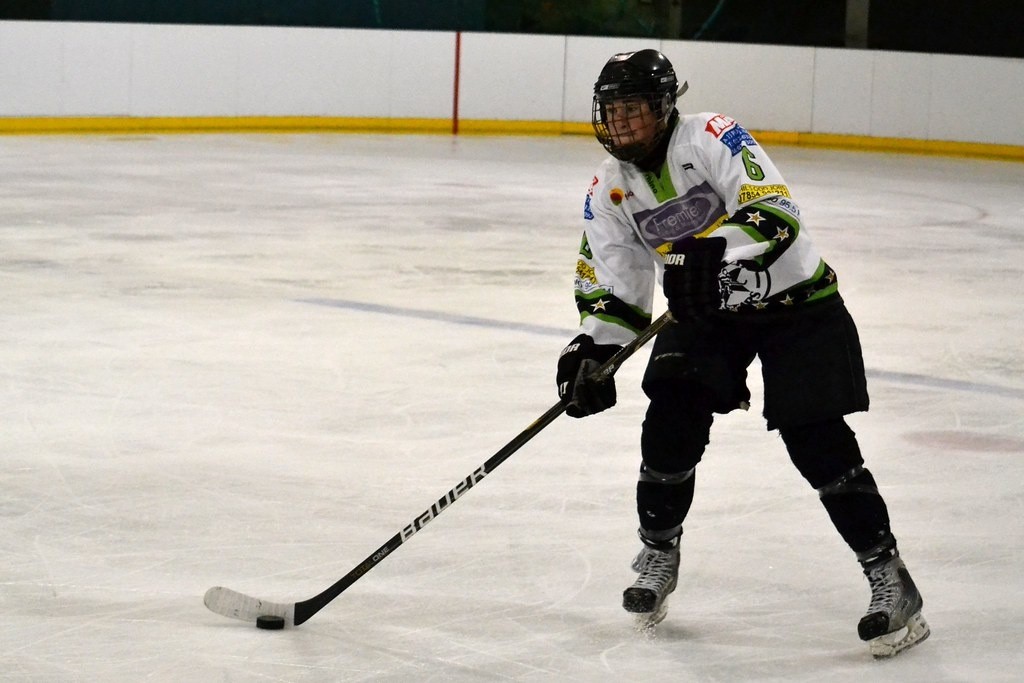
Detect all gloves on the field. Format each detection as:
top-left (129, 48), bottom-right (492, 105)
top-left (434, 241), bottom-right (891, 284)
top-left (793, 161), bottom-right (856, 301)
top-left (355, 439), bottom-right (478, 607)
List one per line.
top-left (557, 333), bottom-right (625, 419)
top-left (663, 236), bottom-right (727, 322)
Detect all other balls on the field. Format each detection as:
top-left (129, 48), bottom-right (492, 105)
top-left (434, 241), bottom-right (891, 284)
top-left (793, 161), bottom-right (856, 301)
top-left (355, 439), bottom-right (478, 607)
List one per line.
top-left (257, 615), bottom-right (286, 631)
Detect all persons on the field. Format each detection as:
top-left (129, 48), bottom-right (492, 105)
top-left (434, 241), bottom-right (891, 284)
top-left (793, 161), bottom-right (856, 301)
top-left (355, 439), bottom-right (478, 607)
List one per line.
top-left (557, 49), bottom-right (930, 659)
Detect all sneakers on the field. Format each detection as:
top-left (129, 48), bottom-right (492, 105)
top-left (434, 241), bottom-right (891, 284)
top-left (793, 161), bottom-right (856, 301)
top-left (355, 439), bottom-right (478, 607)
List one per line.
top-left (858, 535), bottom-right (930, 659)
top-left (622, 529), bottom-right (681, 632)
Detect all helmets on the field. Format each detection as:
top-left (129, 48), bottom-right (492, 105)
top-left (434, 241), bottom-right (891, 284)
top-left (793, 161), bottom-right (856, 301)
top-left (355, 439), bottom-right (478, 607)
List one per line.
top-left (591, 49), bottom-right (678, 169)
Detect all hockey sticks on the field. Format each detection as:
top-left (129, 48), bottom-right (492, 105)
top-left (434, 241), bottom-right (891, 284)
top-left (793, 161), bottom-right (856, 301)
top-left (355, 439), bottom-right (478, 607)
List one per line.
top-left (205, 240), bottom-right (778, 627)
